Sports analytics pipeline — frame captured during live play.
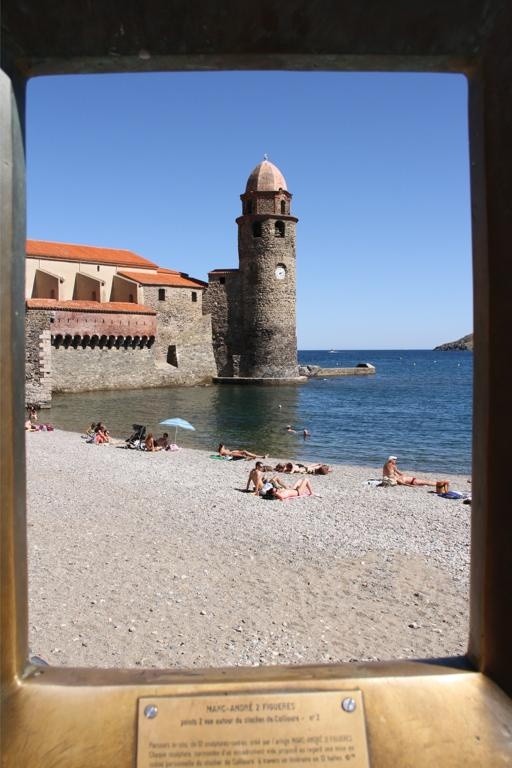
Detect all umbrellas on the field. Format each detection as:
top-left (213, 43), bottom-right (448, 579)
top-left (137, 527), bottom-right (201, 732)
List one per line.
top-left (158, 417), bottom-right (197, 443)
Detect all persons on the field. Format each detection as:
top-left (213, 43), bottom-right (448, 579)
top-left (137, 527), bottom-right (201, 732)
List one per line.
top-left (244, 461), bottom-right (293, 497)
top-left (153, 432), bottom-right (170, 451)
top-left (303, 429), bottom-right (310, 436)
top-left (388, 469), bottom-right (451, 488)
top-left (93, 422), bottom-right (111, 446)
top-left (217, 443), bottom-right (256, 460)
top-left (144, 432), bottom-right (163, 453)
top-left (381, 456), bottom-right (407, 479)
top-left (24, 405), bottom-right (39, 432)
top-left (229, 449), bottom-right (270, 460)
top-left (274, 460), bottom-right (324, 474)
top-left (265, 477), bottom-right (321, 501)
top-left (86, 422), bottom-right (97, 443)
top-left (285, 424), bottom-right (297, 433)
